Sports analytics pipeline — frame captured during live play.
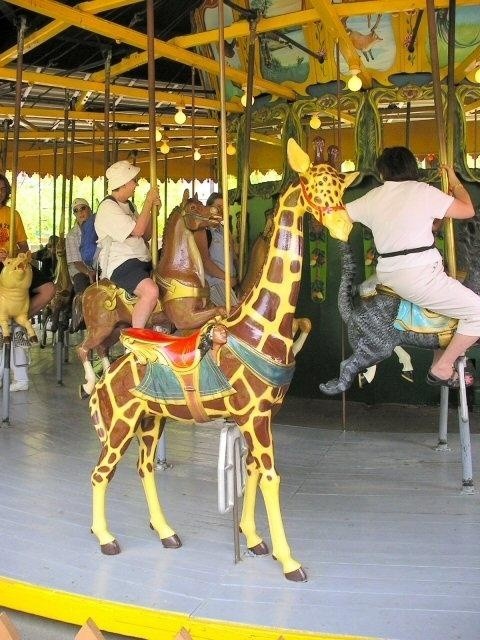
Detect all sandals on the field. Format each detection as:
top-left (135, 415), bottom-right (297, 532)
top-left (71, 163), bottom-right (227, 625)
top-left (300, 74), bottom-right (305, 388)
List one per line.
top-left (13, 326), bottom-right (32, 348)
top-left (425, 367), bottom-right (474, 390)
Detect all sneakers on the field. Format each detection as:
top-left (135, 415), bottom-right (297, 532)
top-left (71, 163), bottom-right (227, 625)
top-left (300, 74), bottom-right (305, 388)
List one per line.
top-left (9, 380), bottom-right (28, 392)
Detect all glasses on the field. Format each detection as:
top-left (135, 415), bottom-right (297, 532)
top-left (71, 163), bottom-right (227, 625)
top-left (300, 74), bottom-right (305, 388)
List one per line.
top-left (73, 206), bottom-right (87, 213)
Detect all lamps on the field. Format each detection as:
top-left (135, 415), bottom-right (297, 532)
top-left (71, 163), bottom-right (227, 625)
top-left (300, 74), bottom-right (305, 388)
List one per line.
top-left (226, 142), bottom-right (236, 155)
top-left (156, 122), bottom-right (162, 142)
top-left (160, 140), bottom-right (170, 154)
top-left (194, 148), bottom-right (201, 161)
top-left (241, 86), bottom-right (254, 107)
top-left (310, 110), bottom-right (321, 129)
top-left (174, 105), bottom-right (186, 125)
top-left (346, 69), bottom-right (363, 92)
top-left (475, 68), bottom-right (480, 84)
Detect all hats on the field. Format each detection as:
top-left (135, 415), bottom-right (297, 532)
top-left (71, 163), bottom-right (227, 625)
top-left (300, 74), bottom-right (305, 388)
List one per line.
top-left (71, 198), bottom-right (89, 209)
top-left (106, 160), bottom-right (141, 191)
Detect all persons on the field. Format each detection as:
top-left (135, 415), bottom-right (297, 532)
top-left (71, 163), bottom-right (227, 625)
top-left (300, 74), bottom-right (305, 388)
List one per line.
top-left (314, 147), bottom-right (480, 388)
top-left (201, 192), bottom-right (238, 307)
top-left (0, 175), bottom-right (57, 319)
top-left (33, 235), bottom-right (59, 272)
top-left (64, 198), bottom-right (97, 294)
top-left (93, 160), bottom-right (163, 329)
